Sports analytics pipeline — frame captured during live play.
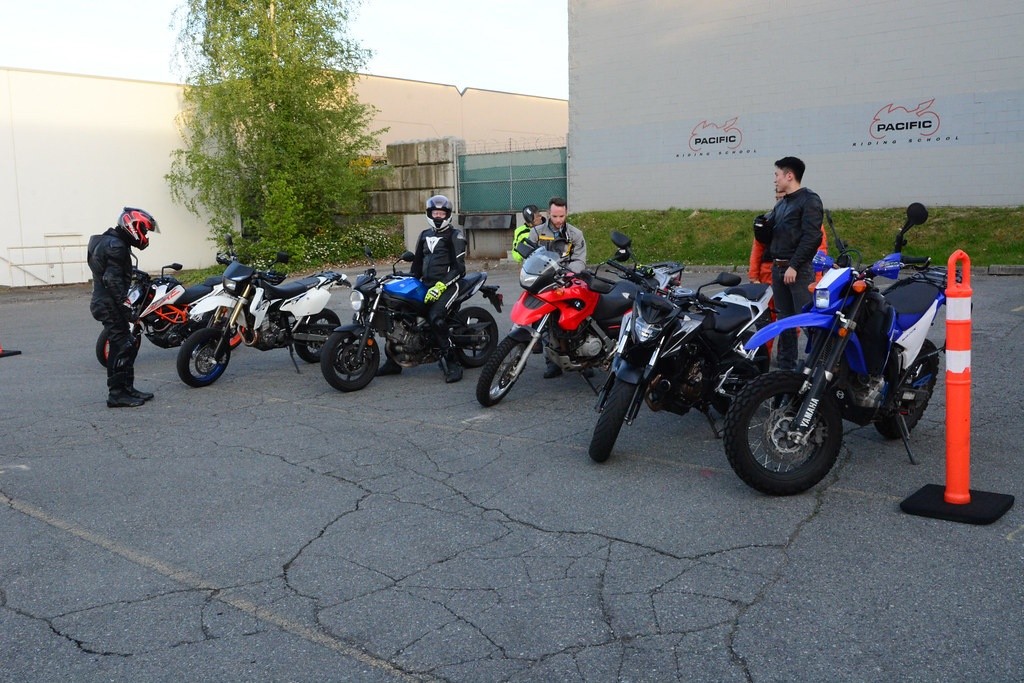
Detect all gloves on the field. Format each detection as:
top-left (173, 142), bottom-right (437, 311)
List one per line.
top-left (753, 214), bottom-right (769, 232)
top-left (120, 302), bottom-right (138, 323)
top-left (424, 281), bottom-right (448, 305)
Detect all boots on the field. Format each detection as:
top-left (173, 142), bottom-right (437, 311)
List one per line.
top-left (375, 359), bottom-right (402, 376)
top-left (107, 372), bottom-right (145, 407)
top-left (125, 366), bottom-right (154, 400)
top-left (443, 347), bottom-right (463, 383)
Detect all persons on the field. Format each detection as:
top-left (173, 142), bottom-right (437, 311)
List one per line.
top-left (753, 156), bottom-right (824, 371)
top-left (376, 195), bottom-right (466, 383)
top-left (511, 205), bottom-right (543, 354)
top-left (748, 181), bottom-right (828, 368)
top-left (526, 197), bottom-right (594, 378)
top-left (87, 206), bottom-right (161, 408)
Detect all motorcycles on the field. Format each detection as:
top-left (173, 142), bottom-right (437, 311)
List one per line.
top-left (319, 245), bottom-right (504, 393)
top-left (583, 230), bottom-right (780, 463)
top-left (94, 250), bottom-right (224, 369)
top-left (713, 202), bottom-right (965, 496)
top-left (169, 232), bottom-right (351, 388)
top-left (471, 206), bottom-right (686, 405)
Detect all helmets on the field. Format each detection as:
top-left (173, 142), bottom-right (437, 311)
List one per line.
top-left (118, 207), bottom-right (155, 251)
top-left (523, 206), bottom-right (539, 223)
top-left (426, 195), bottom-right (452, 230)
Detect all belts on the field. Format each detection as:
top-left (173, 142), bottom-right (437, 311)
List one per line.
top-left (773, 260), bottom-right (789, 267)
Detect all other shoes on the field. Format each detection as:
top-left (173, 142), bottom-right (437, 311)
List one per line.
top-left (584, 367), bottom-right (594, 379)
top-left (533, 342), bottom-right (543, 354)
top-left (543, 362), bottom-right (562, 378)
top-left (766, 397), bottom-right (779, 408)
